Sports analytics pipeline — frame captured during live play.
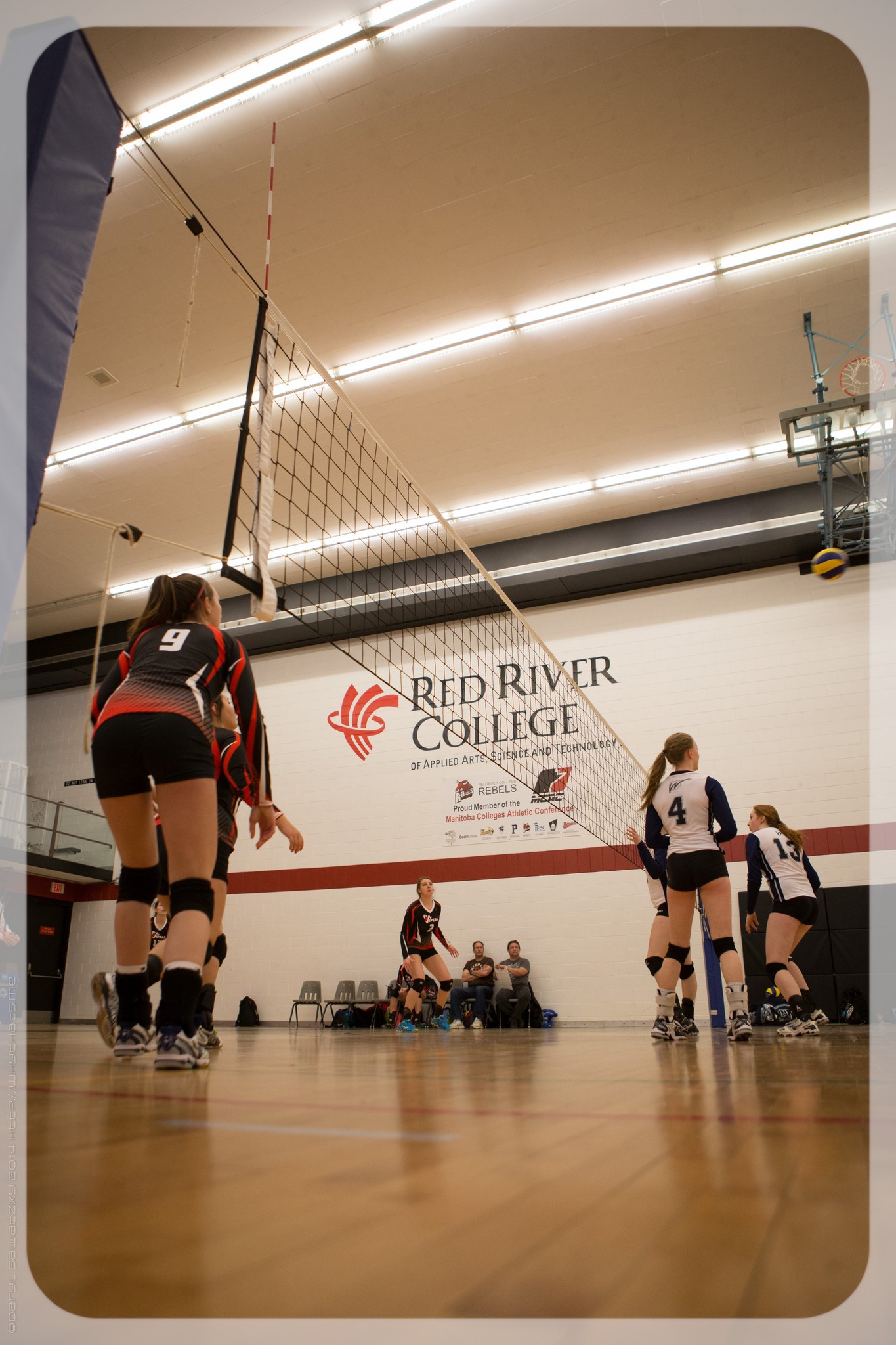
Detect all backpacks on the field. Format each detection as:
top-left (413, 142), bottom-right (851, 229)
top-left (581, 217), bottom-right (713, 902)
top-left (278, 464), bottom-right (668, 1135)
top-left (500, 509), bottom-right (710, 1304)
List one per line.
top-left (235, 996), bottom-right (261, 1028)
top-left (470, 1002), bottom-right (499, 1028)
top-left (424, 974), bottom-right (439, 999)
top-left (541, 1009), bottom-right (558, 1028)
top-left (522, 981), bottom-right (543, 1029)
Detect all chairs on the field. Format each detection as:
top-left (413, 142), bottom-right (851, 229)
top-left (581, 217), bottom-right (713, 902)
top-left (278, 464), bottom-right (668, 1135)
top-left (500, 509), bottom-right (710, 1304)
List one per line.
top-left (499, 981), bottom-right (530, 1028)
top-left (288, 980), bottom-right (324, 1028)
top-left (318, 979), bottom-right (491, 1029)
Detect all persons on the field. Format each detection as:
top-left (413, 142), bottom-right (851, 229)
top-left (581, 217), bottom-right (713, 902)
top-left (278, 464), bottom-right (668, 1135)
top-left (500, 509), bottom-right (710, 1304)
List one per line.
top-left (745, 806), bottom-right (830, 1037)
top-left (87, 573), bottom-right (304, 1069)
top-left (382, 877), bottom-right (532, 1033)
top-left (626, 732), bottom-right (754, 1040)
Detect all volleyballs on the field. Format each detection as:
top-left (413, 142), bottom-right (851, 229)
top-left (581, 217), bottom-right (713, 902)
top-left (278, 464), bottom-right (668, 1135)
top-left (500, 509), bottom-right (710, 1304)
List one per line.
top-left (810, 547), bottom-right (849, 581)
top-left (766, 987), bottom-right (780, 998)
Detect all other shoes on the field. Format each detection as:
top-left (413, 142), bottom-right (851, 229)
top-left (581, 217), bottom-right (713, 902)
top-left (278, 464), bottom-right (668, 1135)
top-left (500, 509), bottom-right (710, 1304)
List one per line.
top-left (509, 1018), bottom-right (524, 1029)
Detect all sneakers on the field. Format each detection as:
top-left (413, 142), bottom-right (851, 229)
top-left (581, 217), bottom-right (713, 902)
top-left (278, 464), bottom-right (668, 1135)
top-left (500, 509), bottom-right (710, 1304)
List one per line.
top-left (112, 1021), bottom-right (163, 1057)
top-left (90, 970), bottom-right (121, 1048)
top-left (726, 1015), bottom-right (755, 1041)
top-left (413, 1021), bottom-right (420, 1028)
top-left (200, 1025), bottom-right (224, 1049)
top-left (673, 1014), bottom-right (700, 1036)
top-left (776, 1017), bottom-right (821, 1036)
top-left (396, 1019), bottom-right (418, 1033)
top-left (431, 1015), bottom-right (453, 1030)
top-left (811, 1009), bottom-right (829, 1026)
top-left (470, 1017), bottom-right (484, 1029)
top-left (449, 1019), bottom-right (465, 1029)
top-left (651, 1016), bottom-right (689, 1040)
top-left (381, 1021), bottom-right (396, 1028)
top-left (153, 1024), bottom-right (210, 1069)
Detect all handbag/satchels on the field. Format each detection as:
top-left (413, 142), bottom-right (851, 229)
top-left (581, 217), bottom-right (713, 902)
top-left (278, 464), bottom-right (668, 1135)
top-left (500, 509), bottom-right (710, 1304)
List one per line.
top-left (755, 998), bottom-right (797, 1025)
top-left (324, 1007), bottom-right (366, 1028)
top-left (364, 1003), bottom-right (390, 1027)
top-left (840, 986), bottom-right (868, 1026)
